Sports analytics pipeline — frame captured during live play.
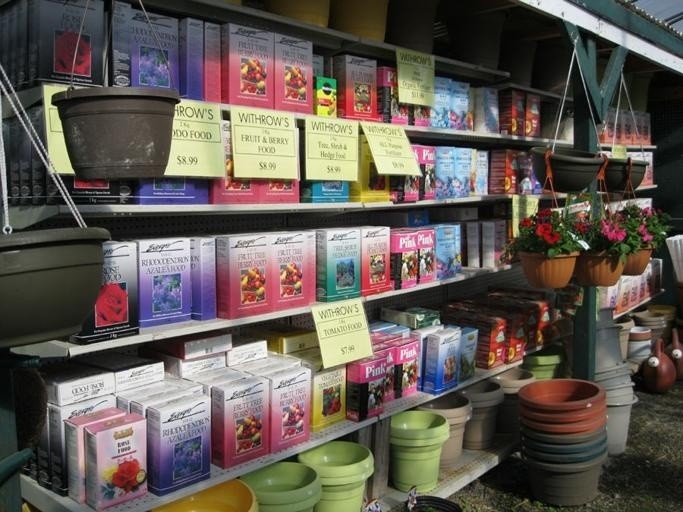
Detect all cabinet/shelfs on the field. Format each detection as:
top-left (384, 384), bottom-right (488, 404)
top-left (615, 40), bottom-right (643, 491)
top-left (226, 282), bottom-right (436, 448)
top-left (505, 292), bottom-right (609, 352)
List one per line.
top-left (0, 0), bottom-right (683, 512)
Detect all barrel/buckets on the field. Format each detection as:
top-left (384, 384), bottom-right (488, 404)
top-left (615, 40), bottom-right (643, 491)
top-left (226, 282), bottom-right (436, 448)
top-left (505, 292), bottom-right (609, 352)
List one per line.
top-left (555, 324), bottom-right (626, 374)
top-left (605, 384), bottom-right (636, 402)
top-left (298, 440), bottom-right (375, 512)
top-left (150, 479), bottom-right (261, 512)
top-left (389, 409), bottom-right (450, 492)
top-left (632, 306), bottom-right (675, 345)
top-left (422, 393), bottom-right (472, 461)
top-left (551, 306), bottom-right (617, 334)
top-left (464, 381), bottom-right (503, 450)
top-left (595, 368), bottom-right (636, 383)
top-left (524, 355), bottom-right (560, 380)
top-left (593, 363), bottom-right (627, 378)
top-left (618, 317), bottom-right (635, 363)
top-left (242, 461), bottom-right (323, 511)
top-left (606, 396), bottom-right (641, 456)
top-left (494, 369), bottom-right (535, 395)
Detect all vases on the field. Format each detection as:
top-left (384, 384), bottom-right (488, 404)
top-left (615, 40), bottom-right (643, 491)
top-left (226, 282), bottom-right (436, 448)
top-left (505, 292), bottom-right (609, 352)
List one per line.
top-left (622, 243), bottom-right (653, 275)
top-left (532, 147), bottom-right (603, 193)
top-left (518, 251), bottom-right (580, 289)
top-left (0, 225), bottom-right (112, 348)
top-left (51, 86), bottom-right (181, 182)
top-left (154, 442), bottom-right (374, 512)
top-left (593, 305), bottom-right (677, 454)
top-left (390, 355), bottom-right (561, 494)
top-left (579, 249), bottom-right (625, 286)
top-left (604, 158), bottom-right (649, 193)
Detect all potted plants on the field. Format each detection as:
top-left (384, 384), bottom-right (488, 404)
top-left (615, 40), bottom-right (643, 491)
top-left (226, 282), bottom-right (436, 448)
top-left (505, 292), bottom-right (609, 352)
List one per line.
top-left (517, 378), bottom-right (608, 506)
